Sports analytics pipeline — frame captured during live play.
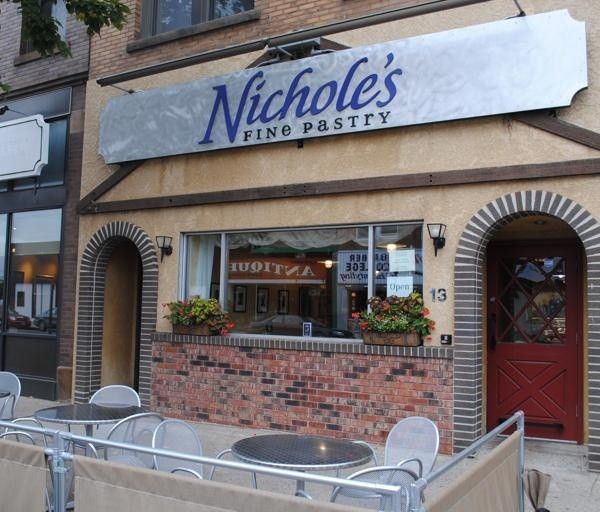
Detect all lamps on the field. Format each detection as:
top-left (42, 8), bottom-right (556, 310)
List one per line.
top-left (426, 222), bottom-right (448, 257)
top-left (156, 235), bottom-right (174, 262)
top-left (316, 246), bottom-right (343, 268)
top-left (376, 238), bottom-right (407, 254)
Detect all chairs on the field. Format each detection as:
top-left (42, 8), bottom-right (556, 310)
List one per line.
top-left (328, 327), bottom-right (356, 339)
top-left (0, 370), bottom-right (75, 455)
top-left (86, 413), bottom-right (165, 471)
top-left (295, 466), bottom-right (425, 511)
top-left (335, 416), bottom-right (440, 503)
top-left (152, 419), bottom-right (257, 490)
top-left (85, 385), bottom-right (142, 457)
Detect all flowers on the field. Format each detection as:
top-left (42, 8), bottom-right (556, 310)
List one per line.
top-left (351, 296), bottom-right (436, 342)
top-left (161, 296), bottom-right (235, 335)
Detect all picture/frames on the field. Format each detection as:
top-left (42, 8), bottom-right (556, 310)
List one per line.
top-left (276, 289), bottom-right (290, 314)
top-left (232, 284), bottom-right (248, 312)
top-left (256, 286), bottom-right (270, 313)
top-left (209, 282), bottom-right (220, 303)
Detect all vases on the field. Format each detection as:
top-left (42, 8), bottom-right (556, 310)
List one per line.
top-left (359, 331), bottom-right (424, 346)
top-left (172, 323), bottom-right (210, 336)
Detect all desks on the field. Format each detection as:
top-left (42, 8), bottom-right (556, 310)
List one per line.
top-left (33, 403), bottom-right (144, 458)
top-left (232, 434), bottom-right (373, 498)
